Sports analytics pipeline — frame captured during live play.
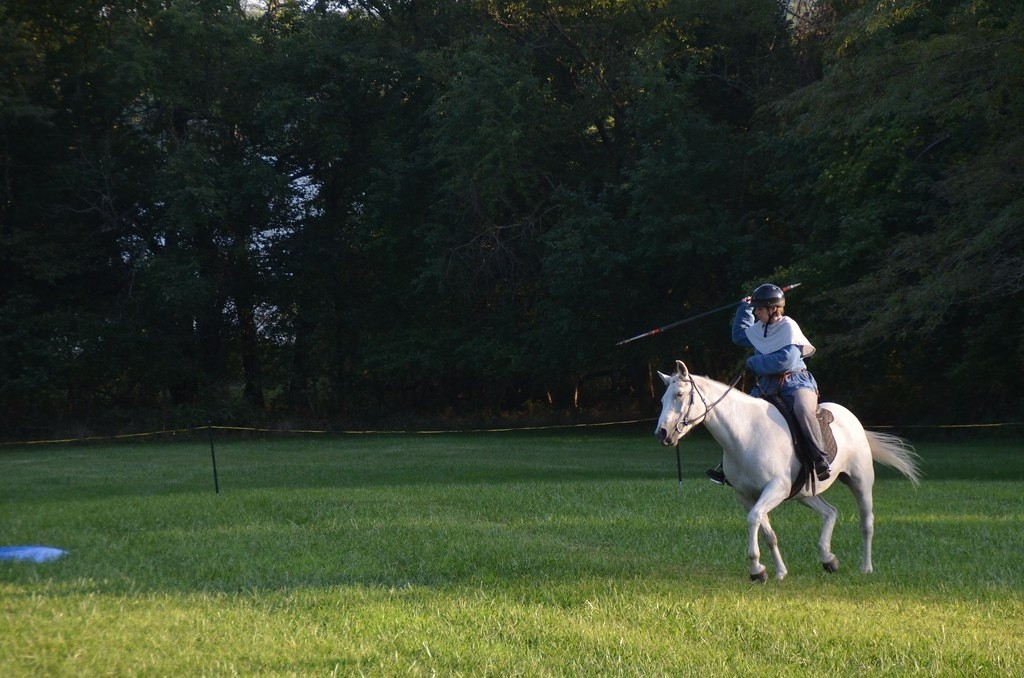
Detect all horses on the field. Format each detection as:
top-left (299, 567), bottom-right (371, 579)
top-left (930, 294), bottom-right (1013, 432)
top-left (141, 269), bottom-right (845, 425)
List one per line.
top-left (654, 360), bottom-right (928, 583)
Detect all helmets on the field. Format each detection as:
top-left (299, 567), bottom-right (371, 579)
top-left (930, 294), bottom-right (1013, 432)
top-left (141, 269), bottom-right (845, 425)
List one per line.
top-left (748, 283), bottom-right (785, 307)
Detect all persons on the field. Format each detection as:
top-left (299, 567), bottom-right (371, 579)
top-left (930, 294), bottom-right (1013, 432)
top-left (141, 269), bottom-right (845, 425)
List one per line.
top-left (706, 283), bottom-right (832, 485)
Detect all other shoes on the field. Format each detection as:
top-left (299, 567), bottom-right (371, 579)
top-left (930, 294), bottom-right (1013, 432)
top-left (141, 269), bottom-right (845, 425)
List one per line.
top-left (707, 466), bottom-right (732, 486)
top-left (817, 459), bottom-right (831, 481)
